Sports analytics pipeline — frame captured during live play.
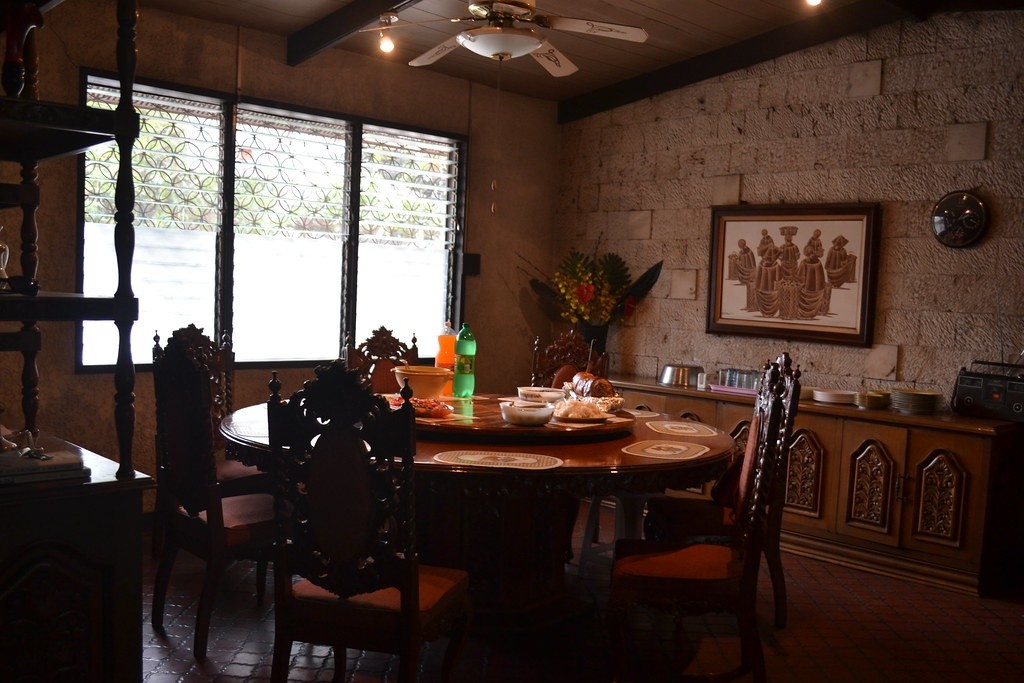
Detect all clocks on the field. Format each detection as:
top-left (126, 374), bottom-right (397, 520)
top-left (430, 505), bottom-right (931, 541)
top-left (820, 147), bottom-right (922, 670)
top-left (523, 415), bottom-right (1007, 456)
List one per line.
top-left (931, 192), bottom-right (989, 249)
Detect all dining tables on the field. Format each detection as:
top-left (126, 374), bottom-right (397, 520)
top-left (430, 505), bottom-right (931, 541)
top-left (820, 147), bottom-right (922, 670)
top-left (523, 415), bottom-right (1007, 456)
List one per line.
top-left (217, 385), bottom-right (739, 644)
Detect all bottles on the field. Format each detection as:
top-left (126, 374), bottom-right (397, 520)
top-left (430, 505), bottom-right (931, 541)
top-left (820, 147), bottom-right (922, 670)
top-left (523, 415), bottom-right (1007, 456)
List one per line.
top-left (434, 322), bottom-right (477, 398)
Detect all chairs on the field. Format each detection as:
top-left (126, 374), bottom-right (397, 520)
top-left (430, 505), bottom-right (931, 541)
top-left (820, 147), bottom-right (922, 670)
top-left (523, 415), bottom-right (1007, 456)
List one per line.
top-left (152, 322), bottom-right (801, 683)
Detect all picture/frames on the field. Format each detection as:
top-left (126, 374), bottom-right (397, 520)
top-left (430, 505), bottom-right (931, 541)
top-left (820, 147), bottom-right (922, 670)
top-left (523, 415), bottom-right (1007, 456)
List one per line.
top-left (705, 201), bottom-right (883, 348)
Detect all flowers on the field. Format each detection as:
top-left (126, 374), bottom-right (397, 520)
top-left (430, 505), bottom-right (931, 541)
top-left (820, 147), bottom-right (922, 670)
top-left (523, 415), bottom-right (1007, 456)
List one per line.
top-left (513, 232), bottom-right (668, 326)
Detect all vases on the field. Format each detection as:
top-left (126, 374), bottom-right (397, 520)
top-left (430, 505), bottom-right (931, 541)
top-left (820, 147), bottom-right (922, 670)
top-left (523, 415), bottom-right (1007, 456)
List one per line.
top-left (574, 325), bottom-right (609, 355)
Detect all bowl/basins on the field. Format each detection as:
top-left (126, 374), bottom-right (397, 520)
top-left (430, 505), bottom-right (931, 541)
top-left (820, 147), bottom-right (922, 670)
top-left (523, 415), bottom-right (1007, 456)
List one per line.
top-left (499, 387), bottom-right (565, 426)
top-left (391, 366), bottom-right (453, 399)
top-left (657, 364), bottom-right (704, 386)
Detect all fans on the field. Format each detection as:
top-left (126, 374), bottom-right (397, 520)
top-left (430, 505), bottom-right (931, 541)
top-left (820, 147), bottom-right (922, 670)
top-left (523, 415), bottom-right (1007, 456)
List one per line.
top-left (360, 0), bottom-right (651, 79)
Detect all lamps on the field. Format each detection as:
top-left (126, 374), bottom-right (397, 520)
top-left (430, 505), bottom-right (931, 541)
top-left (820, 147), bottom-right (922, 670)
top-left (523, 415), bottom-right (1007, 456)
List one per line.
top-left (456, 14), bottom-right (545, 63)
top-left (378, 12), bottom-right (399, 54)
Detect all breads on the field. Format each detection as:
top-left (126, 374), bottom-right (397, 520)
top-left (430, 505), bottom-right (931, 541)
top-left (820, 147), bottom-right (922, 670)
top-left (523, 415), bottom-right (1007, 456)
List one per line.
top-left (572, 372), bottom-right (614, 399)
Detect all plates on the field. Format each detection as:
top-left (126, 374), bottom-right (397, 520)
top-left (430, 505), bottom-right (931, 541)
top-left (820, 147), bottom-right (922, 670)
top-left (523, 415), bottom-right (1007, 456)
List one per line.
top-left (799, 386), bottom-right (944, 414)
top-left (378, 394), bottom-right (455, 417)
top-left (553, 412), bottom-right (617, 423)
top-left (497, 396), bottom-right (520, 401)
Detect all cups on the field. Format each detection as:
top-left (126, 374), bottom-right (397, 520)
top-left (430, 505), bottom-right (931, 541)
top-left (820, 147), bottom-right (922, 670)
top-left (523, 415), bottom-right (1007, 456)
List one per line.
top-left (718, 369), bottom-right (765, 389)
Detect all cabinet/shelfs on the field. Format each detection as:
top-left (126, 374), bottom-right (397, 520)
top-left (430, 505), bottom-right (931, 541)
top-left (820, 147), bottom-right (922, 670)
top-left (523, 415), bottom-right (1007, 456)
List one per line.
top-left (611, 384), bottom-right (1024, 597)
top-left (0, 0), bottom-right (150, 683)
